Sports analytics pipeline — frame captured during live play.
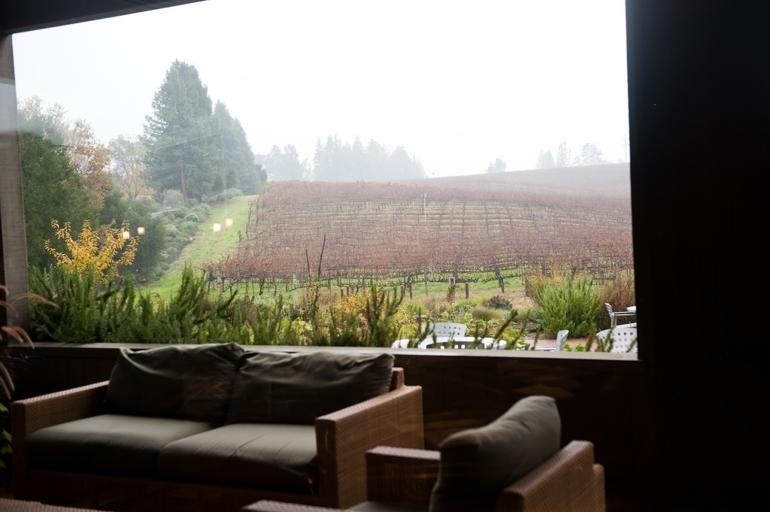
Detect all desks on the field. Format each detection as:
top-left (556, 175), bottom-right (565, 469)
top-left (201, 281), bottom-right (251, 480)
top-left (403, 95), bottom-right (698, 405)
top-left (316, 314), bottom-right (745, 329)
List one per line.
top-left (400, 336), bottom-right (507, 350)
top-left (612, 311), bottom-right (637, 327)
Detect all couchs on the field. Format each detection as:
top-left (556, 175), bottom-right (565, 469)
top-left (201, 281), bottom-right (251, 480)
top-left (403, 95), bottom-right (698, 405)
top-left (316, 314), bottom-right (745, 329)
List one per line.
top-left (9, 341), bottom-right (426, 510)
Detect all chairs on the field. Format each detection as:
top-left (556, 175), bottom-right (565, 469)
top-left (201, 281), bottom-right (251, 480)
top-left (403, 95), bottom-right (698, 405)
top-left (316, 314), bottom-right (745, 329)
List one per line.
top-left (604, 302), bottom-right (618, 328)
top-left (240, 395), bottom-right (608, 510)
top-left (595, 327), bottom-right (638, 353)
top-left (535, 328), bottom-right (569, 352)
